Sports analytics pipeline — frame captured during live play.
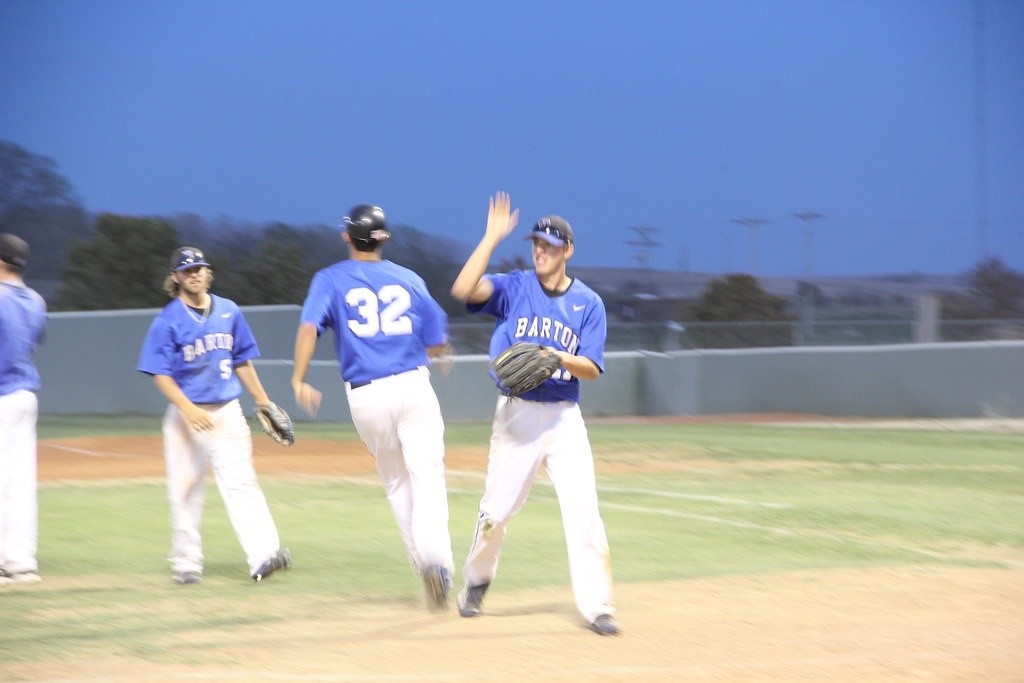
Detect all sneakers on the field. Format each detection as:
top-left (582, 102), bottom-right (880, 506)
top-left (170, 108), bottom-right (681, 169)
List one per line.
top-left (590, 614), bottom-right (619, 636)
top-left (457, 580), bottom-right (491, 618)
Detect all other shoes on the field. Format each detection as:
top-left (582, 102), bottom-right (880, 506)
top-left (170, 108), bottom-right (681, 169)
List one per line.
top-left (172, 570), bottom-right (201, 583)
top-left (251, 551), bottom-right (290, 580)
top-left (424, 565), bottom-right (449, 615)
top-left (0, 569), bottom-right (39, 584)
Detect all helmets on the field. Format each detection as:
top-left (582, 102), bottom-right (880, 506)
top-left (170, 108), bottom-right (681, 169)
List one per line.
top-left (348, 205), bottom-right (385, 242)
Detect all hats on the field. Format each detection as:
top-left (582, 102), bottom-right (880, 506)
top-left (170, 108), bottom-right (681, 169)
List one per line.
top-left (0, 234), bottom-right (28, 270)
top-left (524, 215), bottom-right (573, 246)
top-left (169, 247), bottom-right (210, 272)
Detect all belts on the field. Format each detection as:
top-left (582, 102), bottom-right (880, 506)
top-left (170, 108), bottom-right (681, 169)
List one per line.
top-left (350, 365), bottom-right (418, 389)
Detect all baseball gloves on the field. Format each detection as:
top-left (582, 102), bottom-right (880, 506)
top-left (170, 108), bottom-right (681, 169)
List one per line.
top-left (491, 341), bottom-right (562, 396)
top-left (255, 406), bottom-right (295, 447)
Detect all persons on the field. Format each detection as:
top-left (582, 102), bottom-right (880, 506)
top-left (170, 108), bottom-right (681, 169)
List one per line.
top-left (0, 233), bottom-right (49, 585)
top-left (135, 245), bottom-right (292, 587)
top-left (291, 203), bottom-right (453, 615)
top-left (450, 189), bottom-right (623, 638)
